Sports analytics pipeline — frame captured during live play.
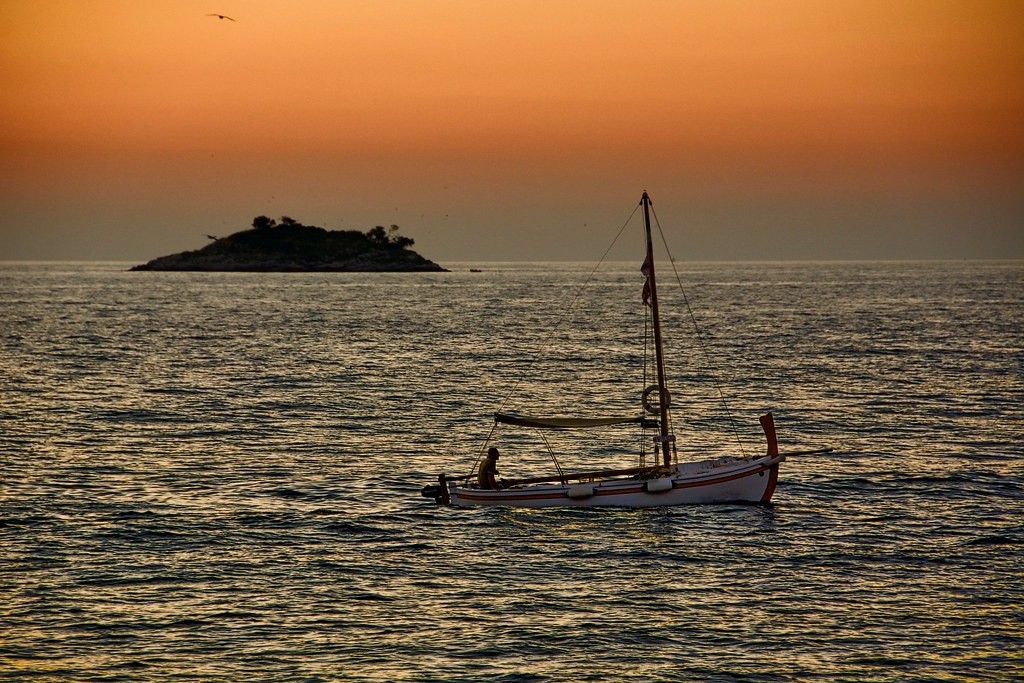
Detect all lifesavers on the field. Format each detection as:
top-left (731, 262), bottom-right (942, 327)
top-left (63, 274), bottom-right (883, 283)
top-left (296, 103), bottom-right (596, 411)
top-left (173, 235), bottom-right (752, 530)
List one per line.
top-left (641, 384), bottom-right (671, 414)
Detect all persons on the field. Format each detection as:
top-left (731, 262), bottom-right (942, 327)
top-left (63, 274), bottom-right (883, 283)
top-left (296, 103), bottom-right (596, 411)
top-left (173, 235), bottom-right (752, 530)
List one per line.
top-left (478, 448), bottom-right (500, 490)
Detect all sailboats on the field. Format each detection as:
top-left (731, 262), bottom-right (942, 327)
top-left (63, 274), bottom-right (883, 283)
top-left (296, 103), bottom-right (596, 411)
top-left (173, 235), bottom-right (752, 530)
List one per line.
top-left (422, 190), bottom-right (787, 510)
